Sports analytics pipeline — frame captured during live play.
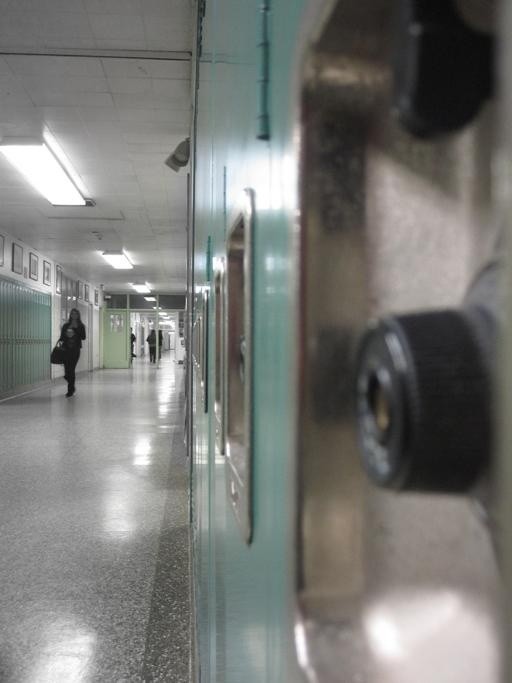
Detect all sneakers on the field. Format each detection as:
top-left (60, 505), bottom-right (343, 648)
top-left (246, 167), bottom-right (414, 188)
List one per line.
top-left (65, 385), bottom-right (76, 398)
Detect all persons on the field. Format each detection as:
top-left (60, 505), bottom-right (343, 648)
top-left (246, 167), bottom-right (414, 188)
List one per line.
top-left (158, 329), bottom-right (163, 359)
top-left (131, 327), bottom-right (136, 363)
top-left (60, 307), bottom-right (87, 396)
top-left (146, 329), bottom-right (156, 362)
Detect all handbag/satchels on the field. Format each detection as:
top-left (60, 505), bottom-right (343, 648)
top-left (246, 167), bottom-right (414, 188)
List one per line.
top-left (50, 338), bottom-right (67, 365)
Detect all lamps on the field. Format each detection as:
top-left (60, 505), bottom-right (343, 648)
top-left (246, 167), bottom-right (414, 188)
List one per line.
top-left (0, 136), bottom-right (176, 329)
